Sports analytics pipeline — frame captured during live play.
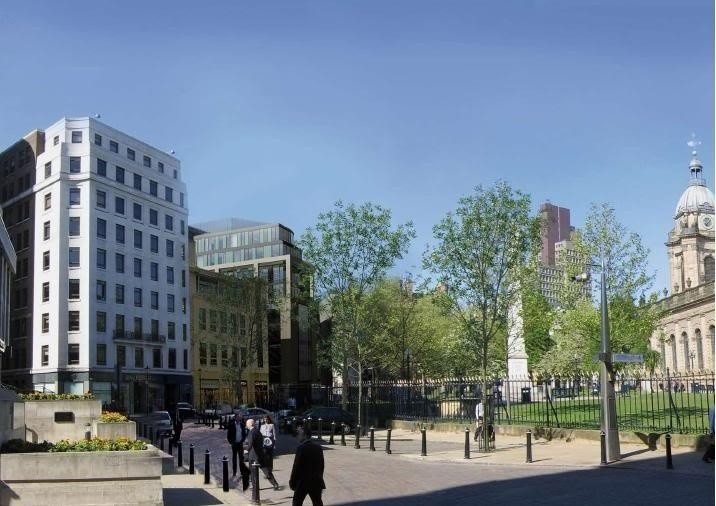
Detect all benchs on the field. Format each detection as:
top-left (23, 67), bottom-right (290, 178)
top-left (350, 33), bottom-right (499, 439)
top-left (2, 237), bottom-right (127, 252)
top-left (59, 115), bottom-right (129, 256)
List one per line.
top-left (552, 387), bottom-right (579, 399)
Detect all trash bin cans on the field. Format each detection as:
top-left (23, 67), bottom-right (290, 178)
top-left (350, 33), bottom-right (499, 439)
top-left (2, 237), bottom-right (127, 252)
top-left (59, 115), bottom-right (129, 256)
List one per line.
top-left (521, 388), bottom-right (530, 404)
top-left (621, 385), bottom-right (631, 397)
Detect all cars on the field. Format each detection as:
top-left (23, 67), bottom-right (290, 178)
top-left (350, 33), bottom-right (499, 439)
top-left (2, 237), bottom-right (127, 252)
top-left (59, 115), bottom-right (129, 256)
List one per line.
top-left (222, 408), bottom-right (274, 431)
top-left (176, 401), bottom-right (192, 419)
top-left (202, 400), bottom-right (232, 417)
top-left (287, 407), bottom-right (354, 435)
top-left (150, 411), bottom-right (174, 437)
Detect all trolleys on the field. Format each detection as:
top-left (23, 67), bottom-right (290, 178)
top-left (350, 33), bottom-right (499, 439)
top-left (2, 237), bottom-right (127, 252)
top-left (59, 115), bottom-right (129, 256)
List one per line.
top-left (476, 417), bottom-right (495, 451)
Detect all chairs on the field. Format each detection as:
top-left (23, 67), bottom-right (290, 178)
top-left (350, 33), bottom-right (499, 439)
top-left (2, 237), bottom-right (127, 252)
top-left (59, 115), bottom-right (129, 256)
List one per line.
top-left (698, 385), bottom-right (714, 394)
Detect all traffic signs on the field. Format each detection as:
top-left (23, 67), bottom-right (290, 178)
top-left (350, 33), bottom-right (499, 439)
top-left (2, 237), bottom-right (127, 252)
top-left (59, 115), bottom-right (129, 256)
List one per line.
top-left (613, 354), bottom-right (643, 361)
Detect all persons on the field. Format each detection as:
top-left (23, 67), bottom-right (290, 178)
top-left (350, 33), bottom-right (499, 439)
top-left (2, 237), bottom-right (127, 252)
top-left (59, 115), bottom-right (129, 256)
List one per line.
top-left (242, 418), bottom-right (279, 493)
top-left (260, 414), bottom-right (277, 476)
top-left (287, 426), bottom-right (326, 506)
top-left (227, 414), bottom-right (246, 478)
top-left (474, 396), bottom-right (485, 442)
